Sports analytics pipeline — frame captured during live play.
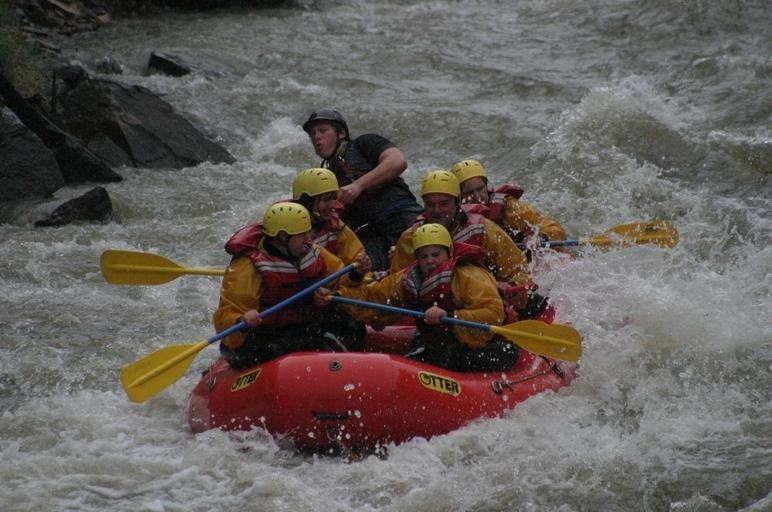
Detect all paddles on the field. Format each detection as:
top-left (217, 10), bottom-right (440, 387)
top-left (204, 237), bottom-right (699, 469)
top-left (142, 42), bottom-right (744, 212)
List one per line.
top-left (513, 221), bottom-right (680, 252)
top-left (324, 295), bottom-right (581, 362)
top-left (100, 250), bottom-right (226, 285)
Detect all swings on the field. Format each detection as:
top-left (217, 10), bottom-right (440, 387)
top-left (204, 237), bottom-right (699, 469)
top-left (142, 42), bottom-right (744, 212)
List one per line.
top-left (120, 263), bottom-right (359, 404)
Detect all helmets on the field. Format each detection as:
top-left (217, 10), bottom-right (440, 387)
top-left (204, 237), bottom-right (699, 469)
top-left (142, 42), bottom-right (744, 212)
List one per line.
top-left (303, 109), bottom-right (349, 137)
top-left (262, 159), bottom-right (486, 256)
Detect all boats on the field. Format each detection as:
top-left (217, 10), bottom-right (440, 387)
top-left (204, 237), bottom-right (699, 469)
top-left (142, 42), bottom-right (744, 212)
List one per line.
top-left (184, 208), bottom-right (587, 464)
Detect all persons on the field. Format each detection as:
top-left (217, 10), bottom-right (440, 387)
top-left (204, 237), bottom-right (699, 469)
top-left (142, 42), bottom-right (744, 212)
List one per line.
top-left (388, 159), bottom-right (565, 260)
top-left (315, 223), bottom-right (518, 373)
top-left (371, 169), bottom-right (538, 331)
top-left (213, 202), bottom-right (371, 373)
top-left (292, 168), bottom-right (365, 265)
top-left (303, 109), bottom-right (424, 270)
